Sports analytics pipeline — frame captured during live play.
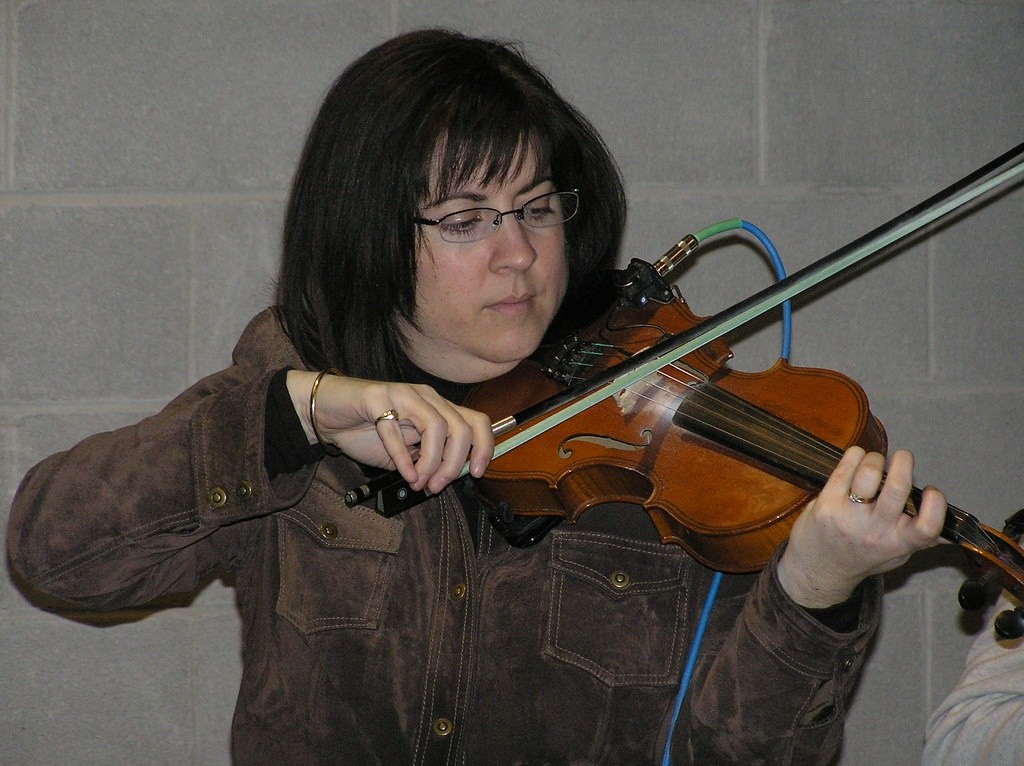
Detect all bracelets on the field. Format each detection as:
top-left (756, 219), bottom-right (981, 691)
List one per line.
top-left (309, 367), bottom-right (346, 457)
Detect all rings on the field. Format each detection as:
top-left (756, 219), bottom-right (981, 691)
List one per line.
top-left (849, 491), bottom-right (875, 505)
top-left (374, 410), bottom-right (399, 427)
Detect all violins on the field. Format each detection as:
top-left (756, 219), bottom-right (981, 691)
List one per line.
top-left (456, 257), bottom-right (1024, 637)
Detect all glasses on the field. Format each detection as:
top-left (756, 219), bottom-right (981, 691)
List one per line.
top-left (413, 187), bottom-right (579, 242)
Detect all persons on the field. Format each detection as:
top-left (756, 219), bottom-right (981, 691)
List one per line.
top-left (922, 530), bottom-right (1024, 765)
top-left (5, 28), bottom-right (946, 766)
